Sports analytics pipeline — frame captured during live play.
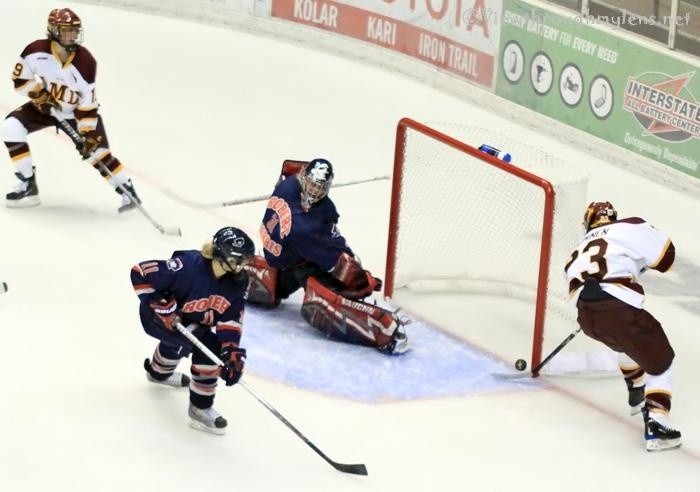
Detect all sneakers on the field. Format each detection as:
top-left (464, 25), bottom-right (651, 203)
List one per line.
top-left (143, 359), bottom-right (191, 387)
top-left (115, 178), bottom-right (141, 213)
top-left (188, 401), bottom-right (228, 428)
top-left (641, 408), bottom-right (681, 440)
top-left (6, 167), bottom-right (39, 200)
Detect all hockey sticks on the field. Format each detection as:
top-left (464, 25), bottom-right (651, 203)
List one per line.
top-left (176, 321), bottom-right (367, 475)
top-left (51, 105), bottom-right (182, 237)
top-left (490, 266), bottom-right (650, 379)
top-left (162, 174), bottom-right (389, 210)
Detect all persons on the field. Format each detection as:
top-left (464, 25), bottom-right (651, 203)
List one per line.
top-left (0, 7), bottom-right (142, 212)
top-left (560, 200), bottom-right (682, 441)
top-left (129, 226), bottom-right (256, 427)
top-left (243, 158), bottom-right (410, 354)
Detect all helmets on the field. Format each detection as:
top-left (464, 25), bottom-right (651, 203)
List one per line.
top-left (46, 7), bottom-right (85, 52)
top-left (302, 157), bottom-right (333, 206)
top-left (581, 200), bottom-right (618, 235)
top-left (212, 226), bottom-right (255, 267)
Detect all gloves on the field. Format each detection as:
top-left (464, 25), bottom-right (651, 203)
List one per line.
top-left (28, 76), bottom-right (62, 115)
top-left (221, 347), bottom-right (247, 385)
top-left (74, 115), bottom-right (101, 160)
top-left (147, 296), bottom-right (180, 332)
